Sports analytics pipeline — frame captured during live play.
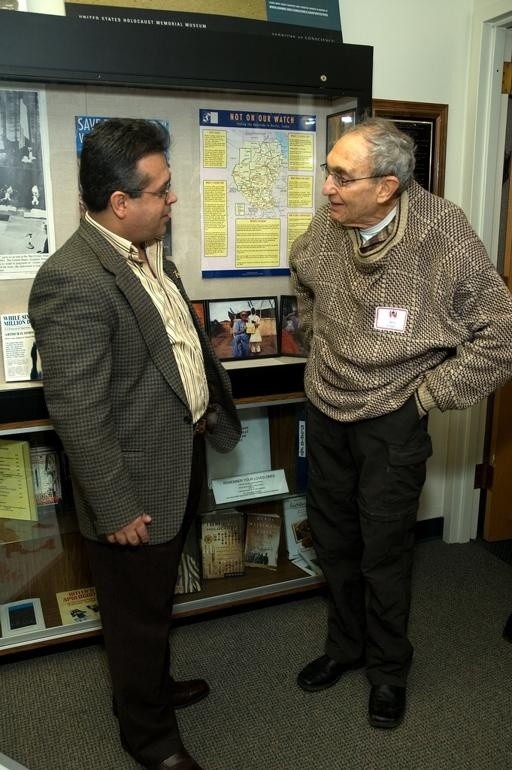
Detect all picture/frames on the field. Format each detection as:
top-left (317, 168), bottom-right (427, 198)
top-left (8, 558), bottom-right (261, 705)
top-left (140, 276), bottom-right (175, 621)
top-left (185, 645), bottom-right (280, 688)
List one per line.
top-left (190, 292), bottom-right (308, 363)
top-left (373, 96), bottom-right (449, 206)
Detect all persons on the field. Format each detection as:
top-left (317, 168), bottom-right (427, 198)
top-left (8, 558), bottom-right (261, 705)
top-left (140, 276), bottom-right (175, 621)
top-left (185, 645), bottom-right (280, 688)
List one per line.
top-left (21, 146), bottom-right (38, 164)
top-left (245, 307), bottom-right (263, 354)
top-left (26, 184), bottom-right (45, 209)
top-left (157, 667), bottom-right (162, 678)
top-left (283, 112), bottom-right (512, 732)
top-left (27, 116), bottom-right (247, 767)
top-left (0, 184), bottom-right (22, 207)
top-left (231, 310), bottom-right (250, 357)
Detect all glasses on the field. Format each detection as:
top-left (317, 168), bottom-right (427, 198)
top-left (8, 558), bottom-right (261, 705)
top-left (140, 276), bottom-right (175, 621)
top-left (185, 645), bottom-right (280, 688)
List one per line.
top-left (121, 183), bottom-right (170, 205)
top-left (320, 163), bottom-right (394, 189)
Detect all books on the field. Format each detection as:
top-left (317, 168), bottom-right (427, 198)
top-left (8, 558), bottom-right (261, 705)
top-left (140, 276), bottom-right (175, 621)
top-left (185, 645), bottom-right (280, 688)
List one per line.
top-left (0, 596), bottom-right (47, 641)
top-left (289, 550), bottom-right (327, 579)
top-left (282, 492), bottom-right (320, 560)
top-left (0, 311), bottom-right (47, 382)
top-left (240, 510), bottom-right (284, 573)
top-left (165, 519), bottom-right (202, 597)
top-left (54, 585), bottom-right (104, 626)
top-left (24, 443), bottom-right (66, 508)
top-left (0, 437), bottom-right (40, 524)
top-left (293, 413), bottom-right (309, 495)
top-left (194, 506), bottom-right (248, 579)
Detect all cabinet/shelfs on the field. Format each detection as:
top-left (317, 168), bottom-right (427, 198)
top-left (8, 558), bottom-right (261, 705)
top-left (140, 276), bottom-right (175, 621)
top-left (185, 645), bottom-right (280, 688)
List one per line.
top-left (1, 390), bottom-right (329, 669)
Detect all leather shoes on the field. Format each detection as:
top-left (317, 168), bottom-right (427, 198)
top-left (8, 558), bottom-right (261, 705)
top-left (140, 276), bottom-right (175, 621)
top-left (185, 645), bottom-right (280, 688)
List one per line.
top-left (368, 686), bottom-right (407, 728)
top-left (155, 749), bottom-right (201, 770)
top-left (171, 678), bottom-right (210, 711)
top-left (298, 648), bottom-right (367, 693)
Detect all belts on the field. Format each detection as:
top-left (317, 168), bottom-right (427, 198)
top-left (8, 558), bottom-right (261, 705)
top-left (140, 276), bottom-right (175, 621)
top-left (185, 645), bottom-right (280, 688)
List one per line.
top-left (192, 418), bottom-right (207, 435)
